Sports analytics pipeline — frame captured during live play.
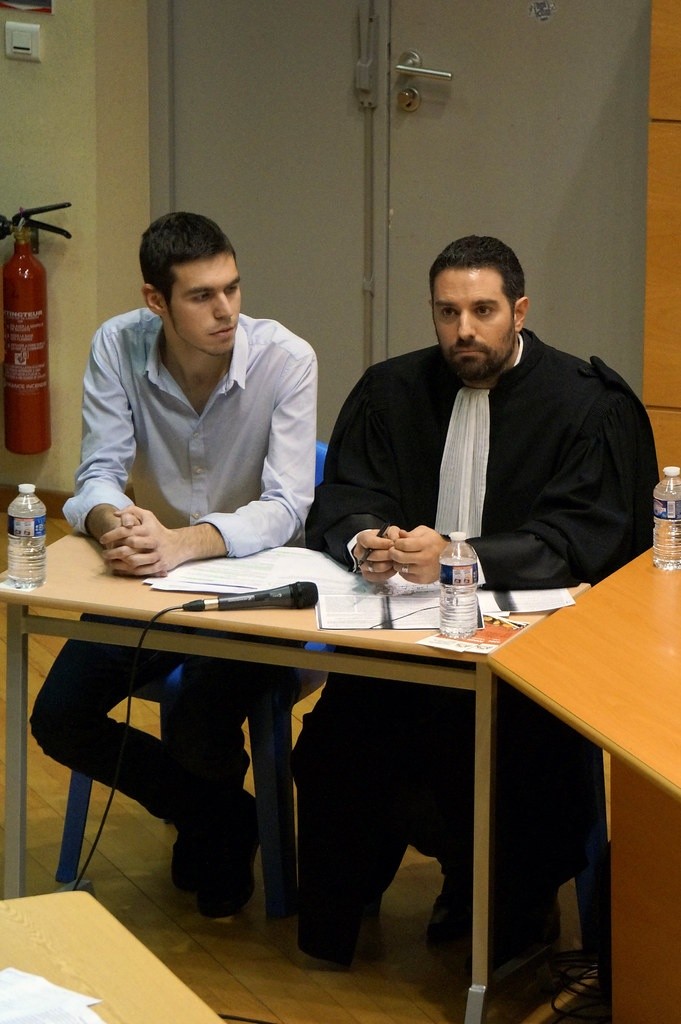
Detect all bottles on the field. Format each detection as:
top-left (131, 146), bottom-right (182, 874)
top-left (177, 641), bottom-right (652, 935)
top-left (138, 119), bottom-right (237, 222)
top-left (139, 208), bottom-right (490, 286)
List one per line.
top-left (439, 531), bottom-right (478, 639)
top-left (7, 484), bottom-right (47, 591)
top-left (653, 467), bottom-right (681, 571)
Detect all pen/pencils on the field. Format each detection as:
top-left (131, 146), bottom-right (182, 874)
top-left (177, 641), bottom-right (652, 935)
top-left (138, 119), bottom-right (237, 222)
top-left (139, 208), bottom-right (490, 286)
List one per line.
top-left (358, 521), bottom-right (392, 568)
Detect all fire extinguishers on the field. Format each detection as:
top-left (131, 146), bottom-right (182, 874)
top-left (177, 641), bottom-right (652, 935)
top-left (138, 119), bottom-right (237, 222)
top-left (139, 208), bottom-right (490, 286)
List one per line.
top-left (1, 201), bottom-right (75, 455)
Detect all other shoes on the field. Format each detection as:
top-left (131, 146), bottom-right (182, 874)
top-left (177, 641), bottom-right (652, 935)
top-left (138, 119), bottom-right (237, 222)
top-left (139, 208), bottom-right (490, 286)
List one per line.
top-left (426, 868), bottom-right (472, 941)
top-left (170, 788), bottom-right (262, 917)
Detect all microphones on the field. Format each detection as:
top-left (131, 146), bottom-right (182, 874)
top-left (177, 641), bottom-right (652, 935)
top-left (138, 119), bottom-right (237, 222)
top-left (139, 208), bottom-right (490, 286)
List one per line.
top-left (181, 582), bottom-right (319, 610)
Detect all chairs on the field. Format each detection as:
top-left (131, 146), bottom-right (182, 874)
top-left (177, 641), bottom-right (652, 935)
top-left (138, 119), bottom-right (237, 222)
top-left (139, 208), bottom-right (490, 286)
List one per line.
top-left (56, 442), bottom-right (329, 914)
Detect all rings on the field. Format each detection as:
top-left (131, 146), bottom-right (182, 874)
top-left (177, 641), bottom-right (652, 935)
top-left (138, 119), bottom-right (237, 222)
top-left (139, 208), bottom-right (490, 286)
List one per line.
top-left (368, 562), bottom-right (375, 575)
top-left (401, 565), bottom-right (410, 576)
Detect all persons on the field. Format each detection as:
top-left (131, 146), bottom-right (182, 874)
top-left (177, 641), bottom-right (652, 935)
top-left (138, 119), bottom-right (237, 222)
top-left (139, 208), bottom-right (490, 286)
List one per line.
top-left (299, 235), bottom-right (659, 966)
top-left (31, 210), bottom-right (319, 917)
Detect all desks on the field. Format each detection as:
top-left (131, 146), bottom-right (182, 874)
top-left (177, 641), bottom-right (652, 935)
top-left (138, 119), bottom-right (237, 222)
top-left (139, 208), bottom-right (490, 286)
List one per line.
top-left (0, 528), bottom-right (593, 1024)
top-left (0, 891), bottom-right (226, 1024)
